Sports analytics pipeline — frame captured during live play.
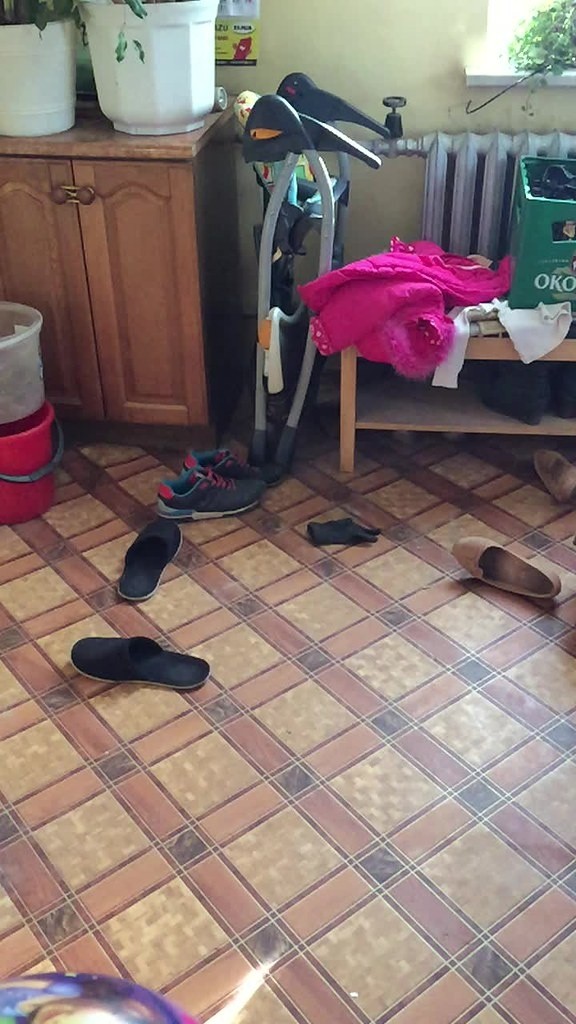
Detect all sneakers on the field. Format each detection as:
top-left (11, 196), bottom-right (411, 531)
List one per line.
top-left (180, 445), bottom-right (264, 481)
top-left (155, 467), bottom-right (266, 517)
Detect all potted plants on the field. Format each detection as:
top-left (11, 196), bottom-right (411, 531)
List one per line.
top-left (0, 0), bottom-right (82, 139)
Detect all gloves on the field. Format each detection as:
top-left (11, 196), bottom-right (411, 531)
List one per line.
top-left (308, 518), bottom-right (382, 546)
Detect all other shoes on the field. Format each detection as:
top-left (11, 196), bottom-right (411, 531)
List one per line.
top-left (452, 536), bottom-right (561, 599)
top-left (479, 367), bottom-right (549, 425)
top-left (549, 364), bottom-right (576, 418)
top-left (534, 449), bottom-right (576, 503)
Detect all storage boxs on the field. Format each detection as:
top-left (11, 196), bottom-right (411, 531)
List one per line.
top-left (504, 155), bottom-right (576, 314)
top-left (0, 299), bottom-right (46, 425)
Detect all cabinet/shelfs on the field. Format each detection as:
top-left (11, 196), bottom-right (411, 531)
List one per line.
top-left (338, 337), bottom-right (576, 472)
top-left (0, 94), bottom-right (257, 451)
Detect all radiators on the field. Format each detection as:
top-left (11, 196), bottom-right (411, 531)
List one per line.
top-left (372, 130), bottom-right (576, 268)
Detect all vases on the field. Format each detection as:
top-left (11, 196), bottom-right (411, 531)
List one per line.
top-left (76, 0), bottom-right (219, 137)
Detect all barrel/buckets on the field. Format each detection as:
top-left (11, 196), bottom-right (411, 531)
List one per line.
top-left (0, 400), bottom-right (63, 524)
top-left (0, 301), bottom-right (45, 425)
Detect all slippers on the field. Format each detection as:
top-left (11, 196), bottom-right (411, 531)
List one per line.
top-left (118, 520), bottom-right (182, 601)
top-left (69, 634), bottom-right (211, 689)
